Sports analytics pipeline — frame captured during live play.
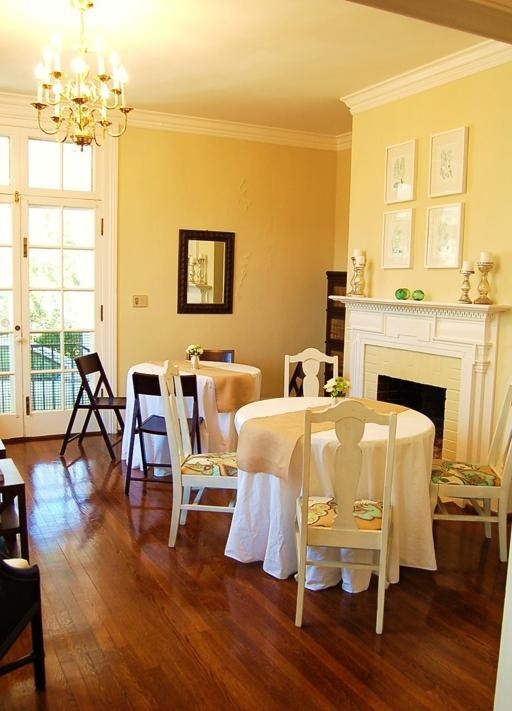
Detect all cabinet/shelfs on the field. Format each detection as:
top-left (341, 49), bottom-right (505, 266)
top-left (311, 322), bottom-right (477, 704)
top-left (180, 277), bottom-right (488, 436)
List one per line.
top-left (322, 268), bottom-right (348, 397)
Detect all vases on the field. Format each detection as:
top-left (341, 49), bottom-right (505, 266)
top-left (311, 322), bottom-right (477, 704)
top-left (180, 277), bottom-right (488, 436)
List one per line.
top-left (190, 356), bottom-right (201, 369)
top-left (331, 397), bottom-right (339, 407)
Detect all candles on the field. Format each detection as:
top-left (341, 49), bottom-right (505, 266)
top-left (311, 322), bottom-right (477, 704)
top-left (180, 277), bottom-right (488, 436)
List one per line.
top-left (479, 250), bottom-right (493, 265)
top-left (354, 247), bottom-right (366, 268)
top-left (188, 253), bottom-right (205, 264)
top-left (462, 258), bottom-right (474, 273)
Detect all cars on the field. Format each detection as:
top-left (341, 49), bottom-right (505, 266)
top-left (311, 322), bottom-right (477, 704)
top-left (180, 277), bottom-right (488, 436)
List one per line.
top-left (0, 339), bottom-right (92, 413)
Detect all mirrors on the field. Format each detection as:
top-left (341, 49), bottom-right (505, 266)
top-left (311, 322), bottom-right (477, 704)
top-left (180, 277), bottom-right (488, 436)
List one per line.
top-left (177, 227), bottom-right (235, 316)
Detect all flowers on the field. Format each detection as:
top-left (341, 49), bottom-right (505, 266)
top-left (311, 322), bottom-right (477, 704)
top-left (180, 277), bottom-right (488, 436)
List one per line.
top-left (323, 374), bottom-right (354, 404)
top-left (186, 345), bottom-right (204, 357)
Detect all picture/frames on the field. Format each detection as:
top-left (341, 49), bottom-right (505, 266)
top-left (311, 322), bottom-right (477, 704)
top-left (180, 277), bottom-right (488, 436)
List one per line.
top-left (425, 125), bottom-right (469, 200)
top-left (423, 203), bottom-right (464, 271)
top-left (380, 207), bottom-right (413, 273)
top-left (383, 136), bottom-right (417, 207)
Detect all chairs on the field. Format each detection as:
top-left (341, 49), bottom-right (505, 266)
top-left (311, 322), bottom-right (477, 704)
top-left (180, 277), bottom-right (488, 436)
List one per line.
top-left (156, 359), bottom-right (241, 550)
top-left (281, 346), bottom-right (339, 399)
top-left (58, 352), bottom-right (130, 463)
top-left (289, 360), bottom-right (305, 397)
top-left (122, 372), bottom-right (204, 501)
top-left (420, 385), bottom-right (512, 566)
top-left (0, 433), bottom-right (50, 695)
top-left (187, 348), bottom-right (235, 364)
top-left (291, 400), bottom-right (402, 637)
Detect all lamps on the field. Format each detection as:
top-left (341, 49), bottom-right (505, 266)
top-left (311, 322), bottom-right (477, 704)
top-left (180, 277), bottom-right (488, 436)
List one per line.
top-left (28, 0), bottom-right (134, 152)
